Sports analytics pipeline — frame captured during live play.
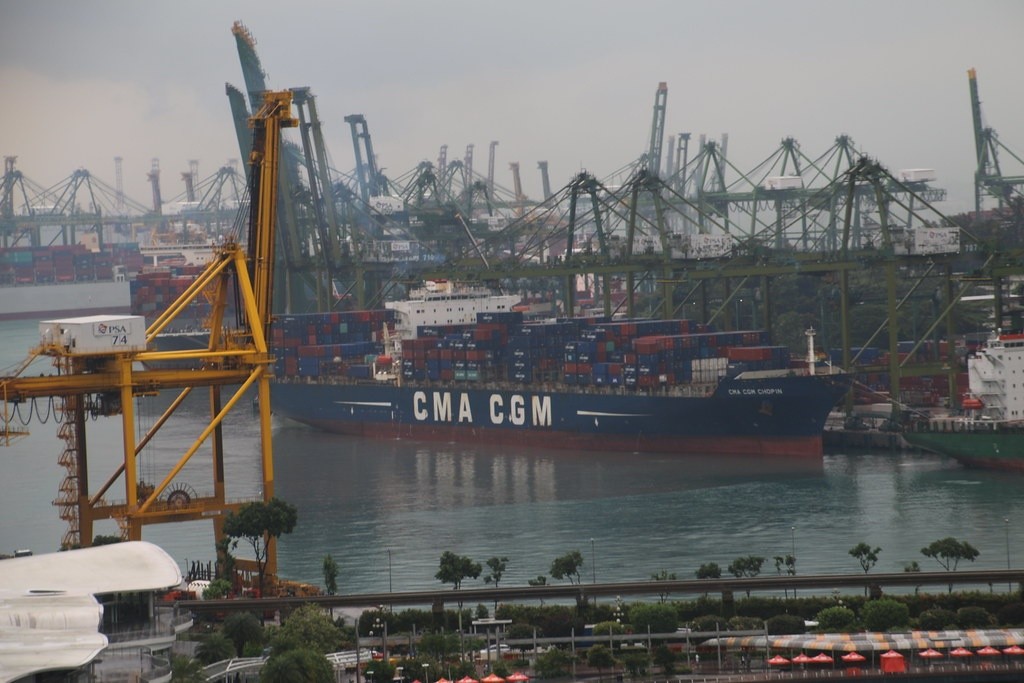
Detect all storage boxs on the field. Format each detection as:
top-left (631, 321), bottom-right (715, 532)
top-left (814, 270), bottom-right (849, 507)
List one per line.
top-left (0, 242), bottom-right (144, 284)
top-left (128, 265), bottom-right (212, 319)
top-left (266, 310), bottom-right (807, 390)
top-left (832, 339), bottom-right (971, 403)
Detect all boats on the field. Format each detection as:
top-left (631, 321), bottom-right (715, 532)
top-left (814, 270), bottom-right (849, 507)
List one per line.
top-left (899, 325), bottom-right (1024, 472)
top-left (960, 399), bottom-right (984, 409)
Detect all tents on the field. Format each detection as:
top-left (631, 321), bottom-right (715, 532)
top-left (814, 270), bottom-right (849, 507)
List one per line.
top-left (766, 645), bottom-right (1024, 674)
top-left (410, 672), bottom-right (530, 683)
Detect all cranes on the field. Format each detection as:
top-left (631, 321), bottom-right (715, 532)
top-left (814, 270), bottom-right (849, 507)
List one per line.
top-left (1, 21), bottom-right (1024, 418)
top-left (0, 86), bottom-right (319, 595)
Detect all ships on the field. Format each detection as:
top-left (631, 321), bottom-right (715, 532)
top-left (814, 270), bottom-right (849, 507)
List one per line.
top-left (266, 279), bottom-right (855, 461)
